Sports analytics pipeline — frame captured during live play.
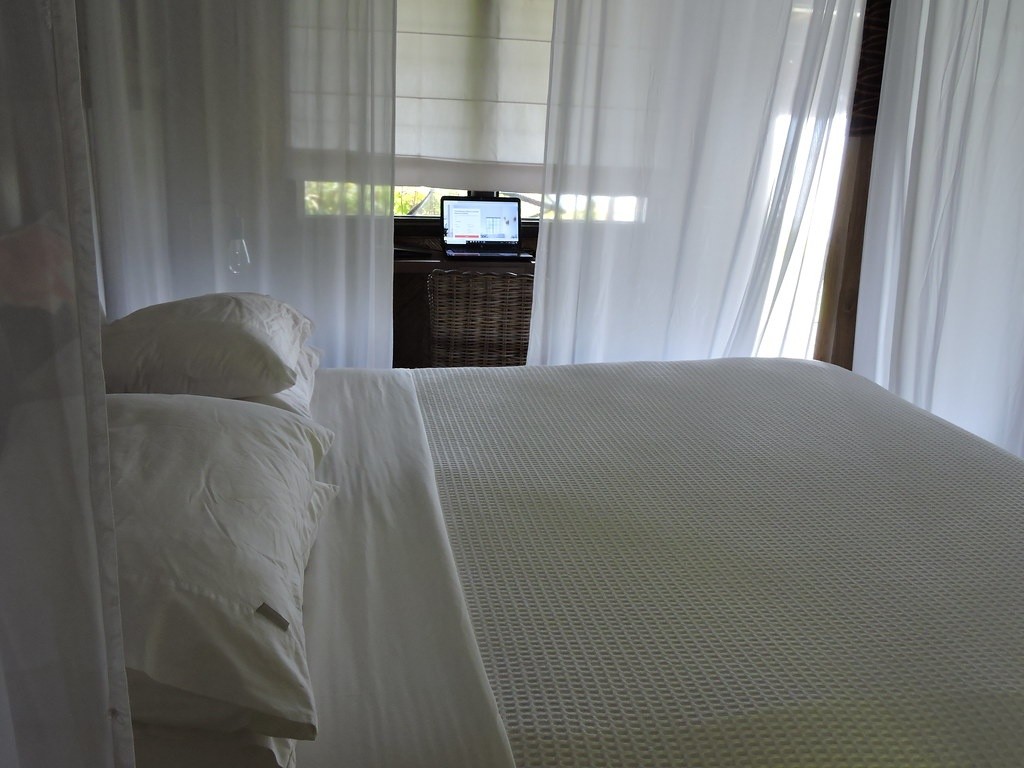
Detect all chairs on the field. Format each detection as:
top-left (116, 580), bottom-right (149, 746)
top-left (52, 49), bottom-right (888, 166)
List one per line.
top-left (427, 269), bottom-right (535, 366)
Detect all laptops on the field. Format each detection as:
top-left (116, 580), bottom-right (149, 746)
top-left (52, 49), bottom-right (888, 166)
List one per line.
top-left (441, 196), bottom-right (533, 262)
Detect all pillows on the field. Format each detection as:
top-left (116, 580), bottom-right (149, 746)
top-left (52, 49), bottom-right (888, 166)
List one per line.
top-left (21, 292), bottom-right (313, 401)
top-left (0, 394), bottom-right (333, 746)
top-left (235, 345), bottom-right (323, 421)
top-left (0, 481), bottom-right (341, 768)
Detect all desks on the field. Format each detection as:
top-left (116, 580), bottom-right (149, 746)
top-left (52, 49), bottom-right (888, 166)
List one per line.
top-left (394, 243), bottom-right (536, 360)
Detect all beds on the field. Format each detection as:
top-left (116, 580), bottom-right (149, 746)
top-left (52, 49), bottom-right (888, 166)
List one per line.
top-left (0, 291), bottom-right (1024, 768)
